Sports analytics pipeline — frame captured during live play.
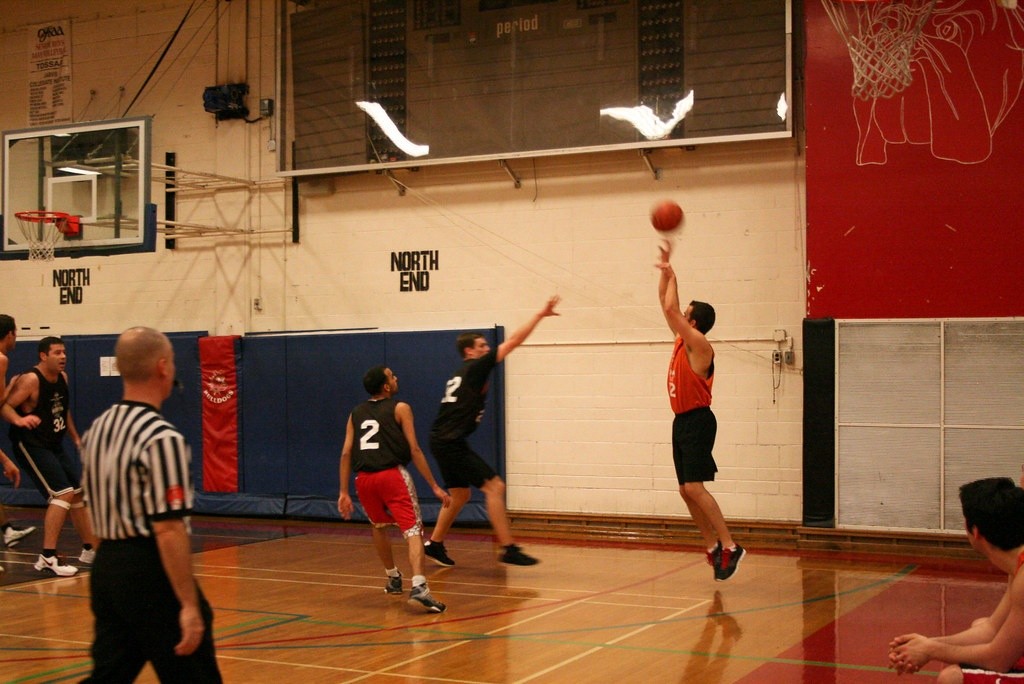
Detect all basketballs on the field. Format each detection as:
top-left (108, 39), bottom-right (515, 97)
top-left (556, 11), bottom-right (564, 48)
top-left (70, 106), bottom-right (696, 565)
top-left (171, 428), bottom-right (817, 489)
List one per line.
top-left (649, 197), bottom-right (684, 233)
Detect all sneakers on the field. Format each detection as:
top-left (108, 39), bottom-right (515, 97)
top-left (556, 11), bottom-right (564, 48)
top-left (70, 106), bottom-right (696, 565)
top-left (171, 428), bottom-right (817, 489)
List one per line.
top-left (716, 542), bottom-right (747, 581)
top-left (79, 546), bottom-right (96, 564)
top-left (407, 582), bottom-right (446, 612)
top-left (497, 543), bottom-right (538, 567)
top-left (424, 539), bottom-right (455, 567)
top-left (3, 526), bottom-right (36, 548)
top-left (706, 540), bottom-right (722, 579)
top-left (384, 571), bottom-right (403, 594)
top-left (34, 553), bottom-right (78, 576)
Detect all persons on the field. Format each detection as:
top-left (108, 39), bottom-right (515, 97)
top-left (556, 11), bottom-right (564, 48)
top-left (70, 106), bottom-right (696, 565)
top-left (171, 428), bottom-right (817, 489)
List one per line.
top-left (423, 296), bottom-right (563, 567)
top-left (0, 313), bottom-right (99, 577)
top-left (337, 366), bottom-right (450, 614)
top-left (888, 465), bottom-right (1024, 684)
top-left (657, 242), bottom-right (745, 581)
top-left (75, 325), bottom-right (224, 683)
